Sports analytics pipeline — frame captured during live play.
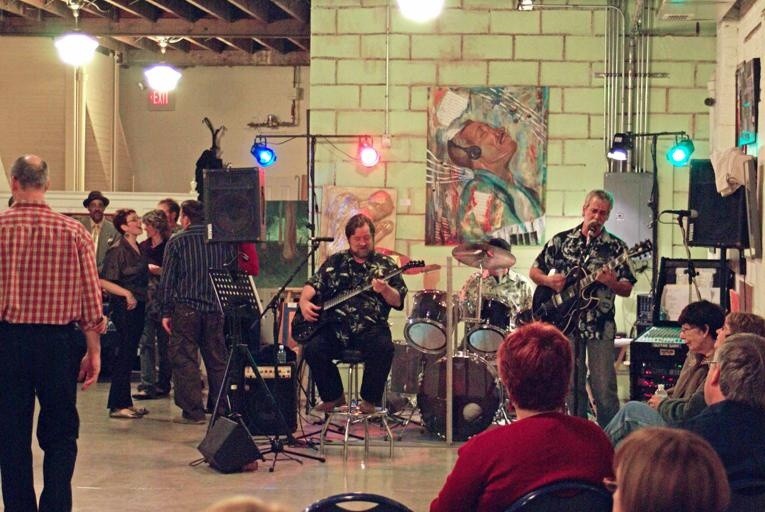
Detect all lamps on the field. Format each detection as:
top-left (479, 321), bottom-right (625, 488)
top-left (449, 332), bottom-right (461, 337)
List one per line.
top-left (251, 136), bottom-right (380, 275)
top-left (143, 36), bottom-right (182, 92)
top-left (607, 131), bottom-right (694, 309)
top-left (55, 0), bottom-right (100, 66)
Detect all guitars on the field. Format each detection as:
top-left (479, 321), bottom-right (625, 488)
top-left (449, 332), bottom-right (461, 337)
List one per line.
top-left (532, 239), bottom-right (654, 334)
top-left (291, 260), bottom-right (424, 344)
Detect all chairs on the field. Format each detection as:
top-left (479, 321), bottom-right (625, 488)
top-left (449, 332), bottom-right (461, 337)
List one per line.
top-left (302, 493), bottom-right (415, 512)
top-left (502, 479), bottom-right (612, 512)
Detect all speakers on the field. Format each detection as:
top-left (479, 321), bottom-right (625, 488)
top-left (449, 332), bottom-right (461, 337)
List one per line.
top-left (203, 167), bottom-right (267, 245)
top-left (685, 159), bottom-right (749, 249)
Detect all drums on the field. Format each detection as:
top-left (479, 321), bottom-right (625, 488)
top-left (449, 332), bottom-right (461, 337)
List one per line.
top-left (416, 350), bottom-right (505, 439)
top-left (465, 298), bottom-right (511, 355)
top-left (403, 289), bottom-right (462, 355)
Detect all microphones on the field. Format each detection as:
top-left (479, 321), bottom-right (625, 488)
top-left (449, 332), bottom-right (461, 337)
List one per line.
top-left (308, 236), bottom-right (334, 241)
top-left (586, 220), bottom-right (599, 247)
top-left (662, 209), bottom-right (697, 218)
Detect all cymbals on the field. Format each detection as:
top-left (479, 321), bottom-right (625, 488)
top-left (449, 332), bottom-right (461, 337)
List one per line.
top-left (452, 242), bottom-right (516, 270)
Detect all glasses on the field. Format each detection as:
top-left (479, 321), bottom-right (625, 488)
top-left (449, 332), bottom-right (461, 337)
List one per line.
top-left (680, 325), bottom-right (698, 334)
top-left (705, 359), bottom-right (718, 368)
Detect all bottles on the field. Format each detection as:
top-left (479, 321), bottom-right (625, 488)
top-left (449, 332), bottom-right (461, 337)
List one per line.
top-left (276, 345), bottom-right (287, 365)
top-left (654, 383), bottom-right (669, 400)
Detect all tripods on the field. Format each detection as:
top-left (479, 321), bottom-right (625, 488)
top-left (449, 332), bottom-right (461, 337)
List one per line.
top-left (206, 294), bottom-right (295, 446)
top-left (246, 253), bottom-right (326, 473)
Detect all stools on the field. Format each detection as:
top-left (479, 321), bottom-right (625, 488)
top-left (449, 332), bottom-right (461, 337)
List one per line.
top-left (320, 350), bottom-right (394, 465)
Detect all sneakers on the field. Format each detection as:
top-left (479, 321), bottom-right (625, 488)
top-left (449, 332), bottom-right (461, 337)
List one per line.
top-left (359, 399), bottom-right (376, 414)
top-left (109, 405), bottom-right (149, 421)
top-left (132, 384), bottom-right (171, 400)
top-left (172, 414), bottom-right (207, 425)
top-left (315, 391), bottom-right (345, 411)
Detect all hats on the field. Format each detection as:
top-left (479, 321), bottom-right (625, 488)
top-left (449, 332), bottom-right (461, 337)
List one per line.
top-left (83, 190), bottom-right (110, 210)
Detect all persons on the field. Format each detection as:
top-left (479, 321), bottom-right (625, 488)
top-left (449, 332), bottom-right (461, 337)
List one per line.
top-left (601, 311), bottom-right (765, 448)
top-left (427, 322), bottom-right (615, 512)
top-left (452, 239), bottom-right (534, 417)
top-left (448, 120), bottom-right (545, 244)
top-left (299, 215), bottom-right (408, 413)
top-left (207, 495), bottom-right (286, 512)
top-left (666, 332), bottom-right (765, 512)
top-left (529, 189), bottom-right (638, 431)
top-left (666, 301), bottom-right (726, 401)
top-left (611, 428), bottom-right (730, 512)
top-left (80, 191), bottom-right (258, 426)
top-left (0, 155), bottom-right (108, 512)
